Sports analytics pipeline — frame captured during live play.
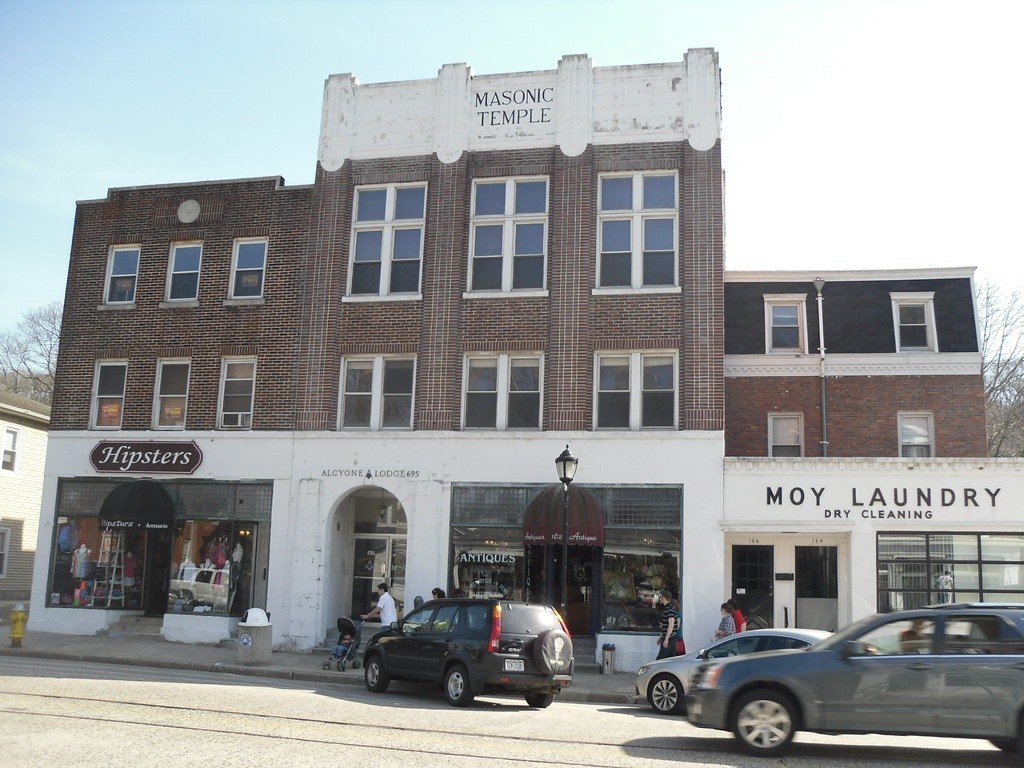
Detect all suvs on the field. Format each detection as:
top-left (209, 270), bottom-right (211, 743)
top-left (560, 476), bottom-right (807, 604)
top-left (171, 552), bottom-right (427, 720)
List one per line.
top-left (361, 597), bottom-right (575, 709)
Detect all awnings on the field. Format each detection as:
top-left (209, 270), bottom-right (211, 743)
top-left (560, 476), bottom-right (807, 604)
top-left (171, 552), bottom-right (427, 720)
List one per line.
top-left (98, 479), bottom-right (175, 533)
top-left (522, 483), bottom-right (604, 548)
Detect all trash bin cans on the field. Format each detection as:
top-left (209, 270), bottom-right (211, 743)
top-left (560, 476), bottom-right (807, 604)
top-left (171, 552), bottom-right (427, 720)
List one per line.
top-left (602, 643), bottom-right (616, 674)
top-left (237, 608), bottom-right (273, 665)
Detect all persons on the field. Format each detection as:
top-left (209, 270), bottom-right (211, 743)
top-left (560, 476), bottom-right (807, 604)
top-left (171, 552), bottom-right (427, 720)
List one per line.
top-left (673, 599), bottom-right (686, 655)
top-left (865, 630), bottom-right (920, 655)
top-left (124, 552), bottom-right (136, 589)
top-left (176, 537), bottom-right (244, 606)
top-left (361, 582), bottom-right (397, 633)
top-left (656, 590), bottom-right (678, 662)
top-left (714, 603), bottom-right (736, 643)
top-left (330, 634), bottom-right (356, 661)
top-left (938, 571), bottom-right (954, 605)
top-left (432, 587), bottom-right (445, 599)
top-left (70, 544), bottom-right (93, 579)
top-left (727, 599), bottom-right (746, 634)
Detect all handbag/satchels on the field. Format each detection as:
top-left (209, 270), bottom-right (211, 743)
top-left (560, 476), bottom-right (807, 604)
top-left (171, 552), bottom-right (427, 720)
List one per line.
top-left (674, 640), bottom-right (685, 655)
top-left (709, 635), bottom-right (727, 655)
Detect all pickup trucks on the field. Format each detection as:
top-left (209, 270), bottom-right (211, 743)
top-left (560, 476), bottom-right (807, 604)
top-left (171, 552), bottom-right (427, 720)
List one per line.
top-left (170, 568), bottom-right (231, 612)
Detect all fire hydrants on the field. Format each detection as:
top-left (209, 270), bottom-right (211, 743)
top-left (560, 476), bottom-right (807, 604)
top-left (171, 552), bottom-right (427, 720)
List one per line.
top-left (8, 603), bottom-right (28, 648)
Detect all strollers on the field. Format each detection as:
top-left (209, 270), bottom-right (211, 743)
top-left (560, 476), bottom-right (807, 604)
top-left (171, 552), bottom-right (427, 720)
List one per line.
top-left (321, 616), bottom-right (367, 672)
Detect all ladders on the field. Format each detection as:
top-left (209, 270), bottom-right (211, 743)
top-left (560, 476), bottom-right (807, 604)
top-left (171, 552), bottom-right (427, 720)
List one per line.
top-left (91, 530), bottom-right (125, 608)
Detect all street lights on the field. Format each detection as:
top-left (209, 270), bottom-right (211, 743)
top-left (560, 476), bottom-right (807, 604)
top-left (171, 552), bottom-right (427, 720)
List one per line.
top-left (554, 444), bottom-right (579, 627)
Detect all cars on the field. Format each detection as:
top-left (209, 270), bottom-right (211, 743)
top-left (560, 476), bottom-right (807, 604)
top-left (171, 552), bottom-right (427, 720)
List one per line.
top-left (683, 606), bottom-right (1023, 757)
top-left (579, 580), bottom-right (681, 630)
top-left (633, 624), bottom-right (880, 716)
